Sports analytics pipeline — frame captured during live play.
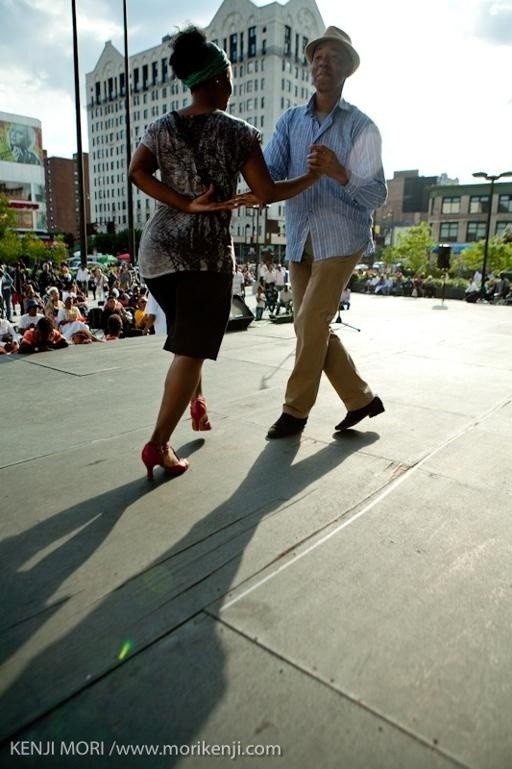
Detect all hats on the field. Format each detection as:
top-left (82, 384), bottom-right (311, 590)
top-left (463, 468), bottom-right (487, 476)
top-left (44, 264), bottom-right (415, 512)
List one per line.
top-left (305, 26), bottom-right (360, 76)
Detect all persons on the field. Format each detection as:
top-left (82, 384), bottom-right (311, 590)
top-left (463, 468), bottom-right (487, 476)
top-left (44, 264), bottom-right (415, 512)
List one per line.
top-left (2, 259), bottom-right (354, 358)
top-left (464, 265), bottom-right (512, 308)
top-left (126, 24), bottom-right (325, 488)
top-left (241, 25), bottom-right (390, 442)
top-left (351, 258), bottom-right (437, 298)
top-left (5, 123), bottom-right (41, 167)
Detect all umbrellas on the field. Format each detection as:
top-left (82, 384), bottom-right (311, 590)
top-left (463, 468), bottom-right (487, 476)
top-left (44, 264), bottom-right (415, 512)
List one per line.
top-left (117, 253), bottom-right (129, 259)
top-left (97, 254), bottom-right (117, 264)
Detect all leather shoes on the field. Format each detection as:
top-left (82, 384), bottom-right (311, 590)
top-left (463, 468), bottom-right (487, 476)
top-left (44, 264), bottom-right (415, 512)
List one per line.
top-left (335, 396), bottom-right (385, 430)
top-left (268, 412), bottom-right (308, 438)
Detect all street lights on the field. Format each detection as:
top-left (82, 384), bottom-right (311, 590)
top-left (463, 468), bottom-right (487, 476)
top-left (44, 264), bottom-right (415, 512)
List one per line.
top-left (471, 171), bottom-right (512, 299)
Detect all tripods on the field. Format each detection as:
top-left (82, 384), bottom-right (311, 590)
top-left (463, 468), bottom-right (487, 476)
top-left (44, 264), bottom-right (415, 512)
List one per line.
top-left (329, 304), bottom-right (360, 332)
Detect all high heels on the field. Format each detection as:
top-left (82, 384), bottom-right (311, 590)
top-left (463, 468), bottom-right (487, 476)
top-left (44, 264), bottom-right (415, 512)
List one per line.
top-left (142, 444), bottom-right (189, 481)
top-left (190, 400), bottom-right (211, 431)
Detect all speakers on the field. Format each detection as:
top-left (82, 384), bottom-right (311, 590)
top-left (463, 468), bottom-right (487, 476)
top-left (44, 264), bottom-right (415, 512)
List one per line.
top-left (225, 294), bottom-right (254, 331)
top-left (437, 244), bottom-right (451, 268)
